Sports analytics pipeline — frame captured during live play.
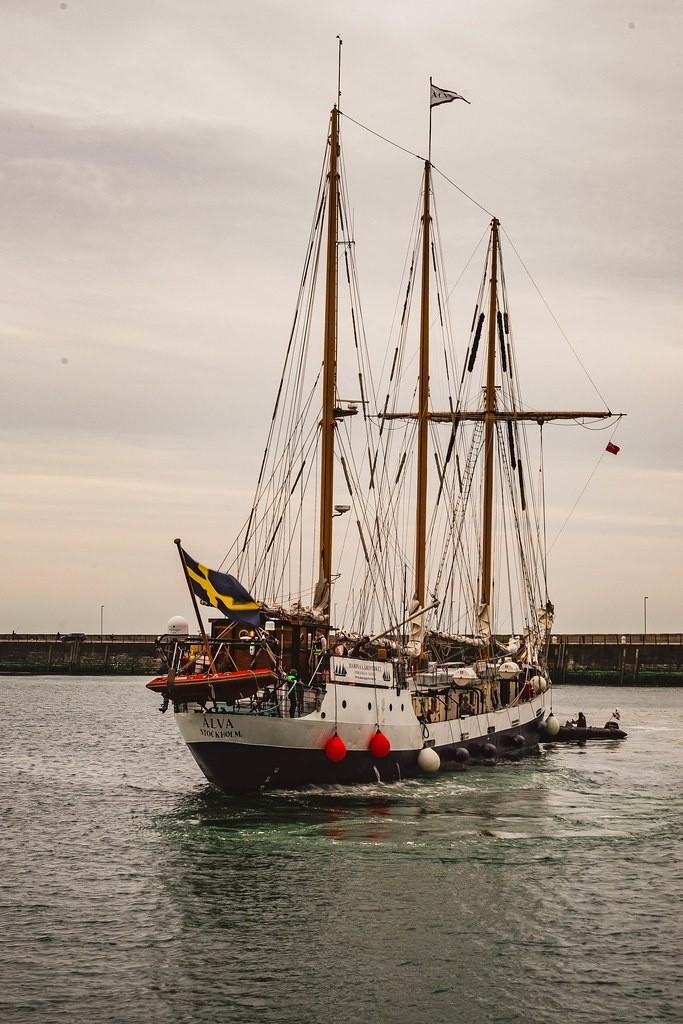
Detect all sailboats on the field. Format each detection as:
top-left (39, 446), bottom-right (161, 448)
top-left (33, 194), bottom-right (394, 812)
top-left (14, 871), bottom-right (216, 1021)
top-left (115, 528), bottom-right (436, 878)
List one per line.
top-left (153, 26), bottom-right (632, 797)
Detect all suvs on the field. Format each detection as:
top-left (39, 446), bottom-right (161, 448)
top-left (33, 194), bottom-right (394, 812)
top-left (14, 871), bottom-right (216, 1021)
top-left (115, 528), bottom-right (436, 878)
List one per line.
top-left (60, 633), bottom-right (87, 642)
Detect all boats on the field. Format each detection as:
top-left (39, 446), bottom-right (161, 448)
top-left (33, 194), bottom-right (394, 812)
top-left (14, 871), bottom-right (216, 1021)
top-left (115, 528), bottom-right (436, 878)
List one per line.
top-left (145, 668), bottom-right (281, 704)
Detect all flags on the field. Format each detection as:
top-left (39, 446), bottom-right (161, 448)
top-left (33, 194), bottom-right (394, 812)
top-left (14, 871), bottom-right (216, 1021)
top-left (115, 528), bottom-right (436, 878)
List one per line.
top-left (612, 708), bottom-right (620, 720)
top-left (180, 547), bottom-right (260, 629)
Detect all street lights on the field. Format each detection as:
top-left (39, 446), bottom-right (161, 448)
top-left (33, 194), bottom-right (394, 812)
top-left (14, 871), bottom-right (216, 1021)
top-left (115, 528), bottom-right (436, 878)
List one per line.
top-left (643, 597), bottom-right (649, 640)
top-left (100, 606), bottom-right (105, 642)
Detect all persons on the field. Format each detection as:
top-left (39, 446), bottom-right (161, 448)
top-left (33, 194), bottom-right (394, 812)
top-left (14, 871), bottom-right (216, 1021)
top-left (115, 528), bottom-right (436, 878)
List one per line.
top-left (459, 696), bottom-right (472, 718)
top-left (572, 712), bottom-right (586, 727)
top-left (352, 636), bottom-right (371, 659)
top-left (287, 669), bottom-right (301, 718)
top-left (314, 628), bottom-right (326, 663)
